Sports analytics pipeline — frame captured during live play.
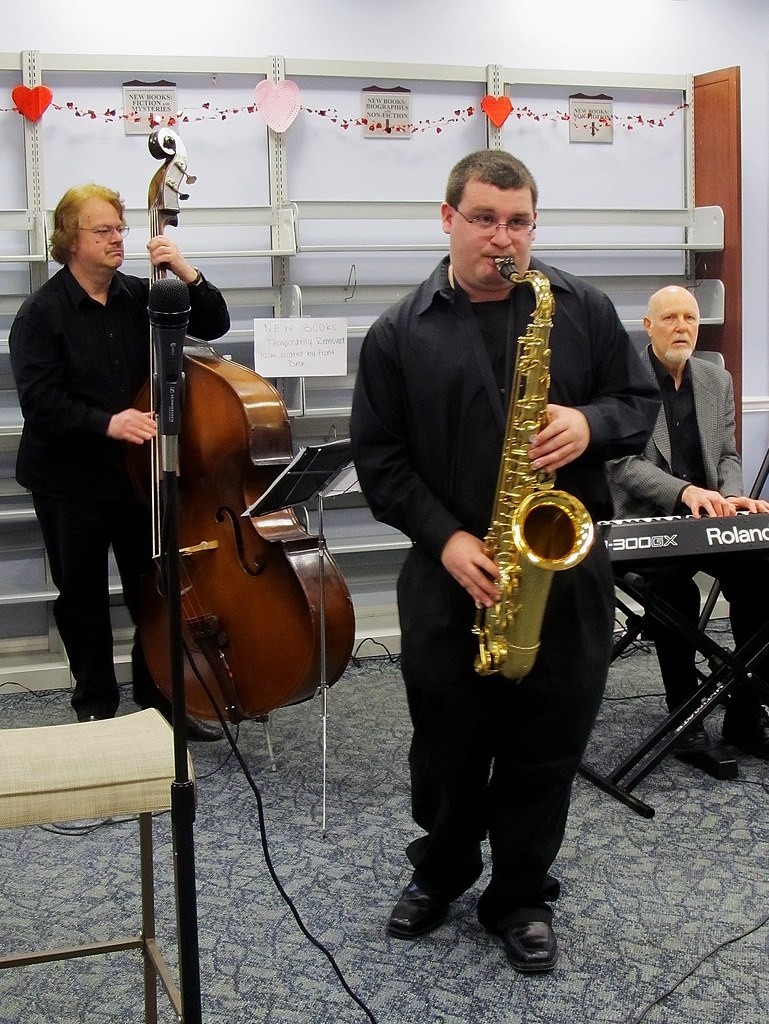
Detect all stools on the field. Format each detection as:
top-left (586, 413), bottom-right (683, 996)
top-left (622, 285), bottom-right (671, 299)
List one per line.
top-left (0, 707), bottom-right (198, 1024)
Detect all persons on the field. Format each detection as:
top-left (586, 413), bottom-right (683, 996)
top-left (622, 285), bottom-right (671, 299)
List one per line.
top-left (8, 182), bottom-right (232, 741)
top-left (347, 146), bottom-right (664, 972)
top-left (599, 284), bottom-right (769, 770)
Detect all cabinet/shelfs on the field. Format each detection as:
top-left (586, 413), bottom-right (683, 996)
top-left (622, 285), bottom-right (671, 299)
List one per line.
top-left (1, 49), bottom-right (727, 695)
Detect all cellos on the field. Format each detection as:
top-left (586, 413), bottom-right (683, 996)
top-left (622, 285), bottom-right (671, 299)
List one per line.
top-left (123, 124), bottom-right (356, 771)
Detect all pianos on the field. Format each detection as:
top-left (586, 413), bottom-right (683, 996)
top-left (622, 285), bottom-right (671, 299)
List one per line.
top-left (597, 512), bottom-right (769, 564)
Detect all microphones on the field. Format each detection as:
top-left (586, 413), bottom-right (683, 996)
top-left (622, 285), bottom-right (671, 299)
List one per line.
top-left (147, 277), bottom-right (192, 501)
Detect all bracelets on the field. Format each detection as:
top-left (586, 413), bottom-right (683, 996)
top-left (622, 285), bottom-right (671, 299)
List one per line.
top-left (184, 267), bottom-right (202, 287)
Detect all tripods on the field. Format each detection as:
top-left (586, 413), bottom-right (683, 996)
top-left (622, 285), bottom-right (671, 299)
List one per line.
top-left (240, 445), bottom-right (412, 839)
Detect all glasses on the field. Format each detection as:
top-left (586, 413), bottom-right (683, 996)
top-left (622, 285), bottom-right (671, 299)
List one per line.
top-left (73, 226), bottom-right (130, 238)
top-left (454, 207), bottom-right (535, 235)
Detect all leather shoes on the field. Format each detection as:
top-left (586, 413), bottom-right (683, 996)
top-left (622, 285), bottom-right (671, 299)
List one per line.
top-left (498, 921), bottom-right (558, 971)
top-left (669, 728), bottom-right (711, 752)
top-left (141, 708), bottom-right (224, 742)
top-left (386, 881), bottom-right (451, 939)
top-left (80, 714), bottom-right (98, 722)
top-left (721, 706), bottom-right (769, 760)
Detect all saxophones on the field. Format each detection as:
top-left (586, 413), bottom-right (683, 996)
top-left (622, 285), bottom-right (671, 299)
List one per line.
top-left (470, 258), bottom-right (595, 680)
